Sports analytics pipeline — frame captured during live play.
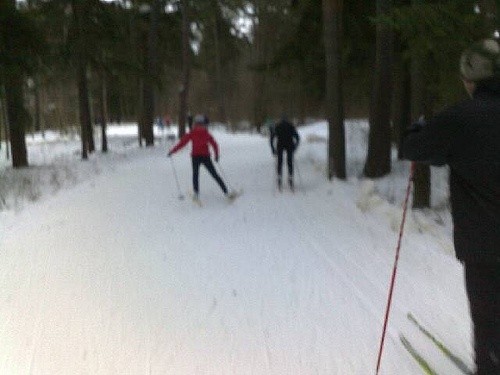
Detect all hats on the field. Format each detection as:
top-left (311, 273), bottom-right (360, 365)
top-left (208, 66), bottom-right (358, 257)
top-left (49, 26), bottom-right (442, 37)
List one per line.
top-left (459, 38), bottom-right (500, 83)
top-left (195, 114), bottom-right (206, 124)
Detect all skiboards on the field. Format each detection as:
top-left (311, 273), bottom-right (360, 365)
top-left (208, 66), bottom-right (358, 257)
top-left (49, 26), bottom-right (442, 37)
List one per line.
top-left (399, 311), bottom-right (475, 375)
top-left (187, 186), bottom-right (244, 208)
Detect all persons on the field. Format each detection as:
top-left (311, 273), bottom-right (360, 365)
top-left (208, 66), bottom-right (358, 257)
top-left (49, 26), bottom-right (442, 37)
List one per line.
top-left (167, 114), bottom-right (233, 203)
top-left (270, 113), bottom-right (300, 192)
top-left (398, 38), bottom-right (499, 375)
